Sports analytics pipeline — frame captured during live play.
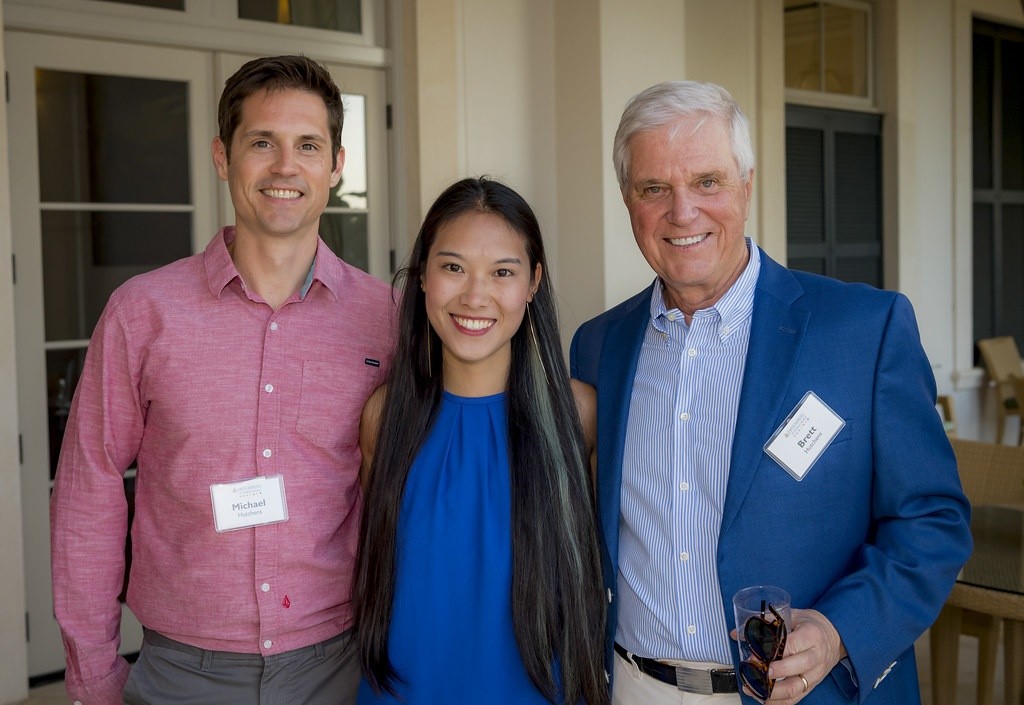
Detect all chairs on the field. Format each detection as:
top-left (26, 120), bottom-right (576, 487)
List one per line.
top-left (978, 336), bottom-right (1024, 447)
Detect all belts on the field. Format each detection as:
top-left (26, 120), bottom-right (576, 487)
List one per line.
top-left (614, 640), bottom-right (738, 694)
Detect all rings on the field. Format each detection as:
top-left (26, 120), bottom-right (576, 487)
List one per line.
top-left (798, 673), bottom-right (810, 695)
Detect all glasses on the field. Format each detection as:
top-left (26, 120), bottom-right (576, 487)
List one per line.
top-left (736, 600), bottom-right (788, 702)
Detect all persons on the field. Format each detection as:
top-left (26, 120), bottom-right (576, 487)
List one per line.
top-left (46, 50), bottom-right (413, 704)
top-left (358, 177), bottom-right (601, 704)
top-left (569, 80), bottom-right (981, 704)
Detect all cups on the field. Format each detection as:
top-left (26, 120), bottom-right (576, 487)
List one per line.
top-left (733, 585), bottom-right (792, 681)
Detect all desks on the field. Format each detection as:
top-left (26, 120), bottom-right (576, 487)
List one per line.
top-left (946, 437), bottom-right (1024, 593)
